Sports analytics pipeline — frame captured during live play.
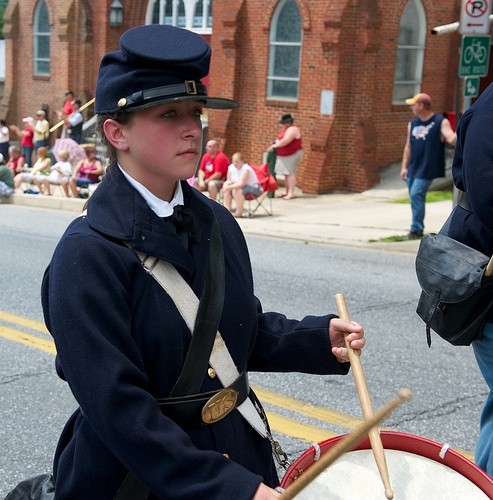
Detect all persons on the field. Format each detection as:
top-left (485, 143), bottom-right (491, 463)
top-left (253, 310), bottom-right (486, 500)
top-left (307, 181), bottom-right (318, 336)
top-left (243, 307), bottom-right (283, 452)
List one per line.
top-left (43, 24), bottom-right (366, 500)
top-left (268, 114), bottom-right (303, 199)
top-left (223, 151), bottom-right (261, 217)
top-left (447, 83), bottom-right (493, 479)
top-left (191, 140), bottom-right (228, 202)
top-left (400, 92), bottom-right (458, 240)
top-left (0, 91), bottom-right (103, 203)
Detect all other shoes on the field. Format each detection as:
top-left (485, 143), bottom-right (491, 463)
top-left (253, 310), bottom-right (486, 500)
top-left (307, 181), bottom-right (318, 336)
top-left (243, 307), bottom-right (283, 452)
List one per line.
top-left (402, 233), bottom-right (423, 239)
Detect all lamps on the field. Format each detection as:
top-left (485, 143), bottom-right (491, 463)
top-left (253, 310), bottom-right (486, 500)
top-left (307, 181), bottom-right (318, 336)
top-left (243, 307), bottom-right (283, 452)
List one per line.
top-left (108, 0), bottom-right (125, 28)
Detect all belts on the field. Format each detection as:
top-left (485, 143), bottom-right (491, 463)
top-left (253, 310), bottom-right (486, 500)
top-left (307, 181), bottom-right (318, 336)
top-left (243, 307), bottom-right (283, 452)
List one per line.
top-left (156, 369), bottom-right (250, 427)
top-left (455, 187), bottom-right (474, 213)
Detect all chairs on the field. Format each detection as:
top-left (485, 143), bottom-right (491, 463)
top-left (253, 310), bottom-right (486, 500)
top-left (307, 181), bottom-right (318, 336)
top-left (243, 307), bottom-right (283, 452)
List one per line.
top-left (231, 163), bottom-right (280, 219)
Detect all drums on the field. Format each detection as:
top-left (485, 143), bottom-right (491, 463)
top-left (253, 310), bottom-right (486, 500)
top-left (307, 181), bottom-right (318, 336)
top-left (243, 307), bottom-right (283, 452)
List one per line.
top-left (278, 427), bottom-right (493, 500)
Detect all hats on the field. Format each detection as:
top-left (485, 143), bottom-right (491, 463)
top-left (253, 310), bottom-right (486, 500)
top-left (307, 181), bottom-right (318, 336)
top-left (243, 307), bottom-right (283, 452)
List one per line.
top-left (279, 114), bottom-right (294, 123)
top-left (94, 25), bottom-right (240, 114)
top-left (36, 110), bottom-right (45, 115)
top-left (0, 153), bottom-right (4, 163)
top-left (23, 117), bottom-right (35, 125)
top-left (405, 93), bottom-right (431, 105)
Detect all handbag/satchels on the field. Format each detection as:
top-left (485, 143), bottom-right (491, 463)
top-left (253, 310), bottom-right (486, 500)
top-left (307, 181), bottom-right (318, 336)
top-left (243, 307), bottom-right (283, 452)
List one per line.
top-left (415, 233), bottom-right (493, 348)
